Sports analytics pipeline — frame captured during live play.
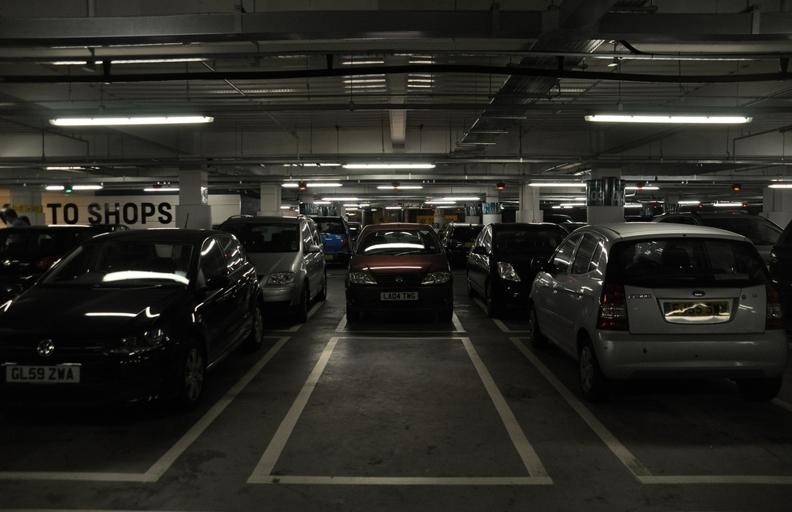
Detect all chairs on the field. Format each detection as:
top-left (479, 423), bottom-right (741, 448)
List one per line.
top-left (241, 231), bottom-right (296, 251)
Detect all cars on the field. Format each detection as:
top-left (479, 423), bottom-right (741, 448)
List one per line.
top-left (310, 216), bottom-right (361, 267)
top-left (437, 223), bottom-right (487, 266)
top-left (0, 224), bottom-right (265, 424)
top-left (344, 222), bottom-right (454, 329)
top-left (526, 221), bottom-right (790, 402)
top-left (651, 206), bottom-right (784, 270)
top-left (465, 223), bottom-right (569, 318)
top-left (0, 223), bottom-right (130, 308)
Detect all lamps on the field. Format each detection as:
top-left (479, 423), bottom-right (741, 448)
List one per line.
top-left (585, 60), bottom-right (754, 125)
top-left (282, 162), bottom-right (480, 207)
top-left (46, 183), bottom-right (206, 195)
top-left (44, 66), bottom-right (216, 128)
top-left (529, 180), bottom-right (792, 210)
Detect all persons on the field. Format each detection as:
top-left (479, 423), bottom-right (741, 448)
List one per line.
top-left (5, 209), bottom-right (30, 228)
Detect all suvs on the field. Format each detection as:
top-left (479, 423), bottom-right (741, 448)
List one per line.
top-left (216, 215), bottom-right (328, 323)
